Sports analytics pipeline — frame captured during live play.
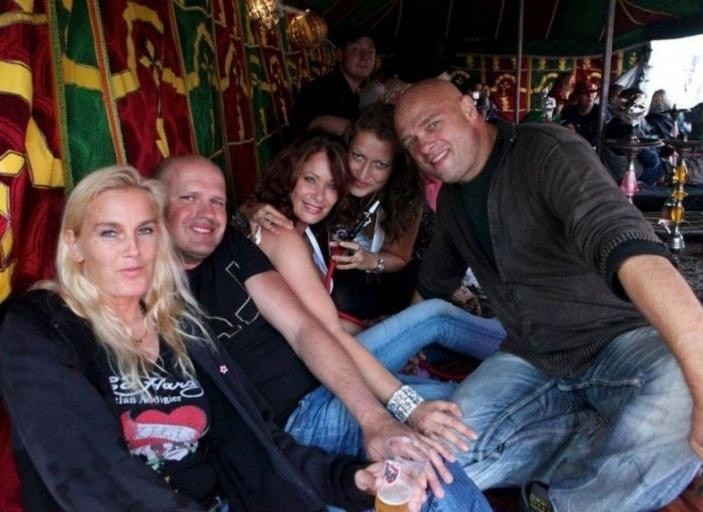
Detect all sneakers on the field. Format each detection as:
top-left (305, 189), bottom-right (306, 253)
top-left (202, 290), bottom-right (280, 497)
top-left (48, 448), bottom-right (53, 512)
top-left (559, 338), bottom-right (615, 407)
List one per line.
top-left (529, 481), bottom-right (553, 512)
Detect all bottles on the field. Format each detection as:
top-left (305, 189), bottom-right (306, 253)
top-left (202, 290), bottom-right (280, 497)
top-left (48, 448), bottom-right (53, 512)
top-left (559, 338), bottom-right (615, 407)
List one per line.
top-left (341, 215), bottom-right (371, 255)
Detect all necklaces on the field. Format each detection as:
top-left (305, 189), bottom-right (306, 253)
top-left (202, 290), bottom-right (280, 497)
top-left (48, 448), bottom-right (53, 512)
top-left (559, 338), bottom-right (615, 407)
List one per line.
top-left (131, 302), bottom-right (149, 345)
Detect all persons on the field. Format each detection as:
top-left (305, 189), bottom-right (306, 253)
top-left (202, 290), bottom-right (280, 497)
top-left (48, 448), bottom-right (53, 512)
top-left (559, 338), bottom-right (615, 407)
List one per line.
top-left (226, 23), bottom-right (507, 455)
top-left (151, 154), bottom-right (493, 511)
top-left (541, 58), bottom-right (701, 188)
top-left (393, 78), bottom-right (702, 511)
top-left (0, 164), bottom-right (428, 512)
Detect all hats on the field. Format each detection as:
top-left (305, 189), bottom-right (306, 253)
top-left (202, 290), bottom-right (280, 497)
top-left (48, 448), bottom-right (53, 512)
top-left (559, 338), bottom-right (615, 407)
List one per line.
top-left (575, 80), bottom-right (598, 94)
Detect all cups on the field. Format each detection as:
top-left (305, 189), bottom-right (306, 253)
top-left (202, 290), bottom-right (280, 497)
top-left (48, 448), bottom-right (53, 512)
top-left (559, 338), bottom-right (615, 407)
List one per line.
top-left (377, 435), bottom-right (432, 492)
